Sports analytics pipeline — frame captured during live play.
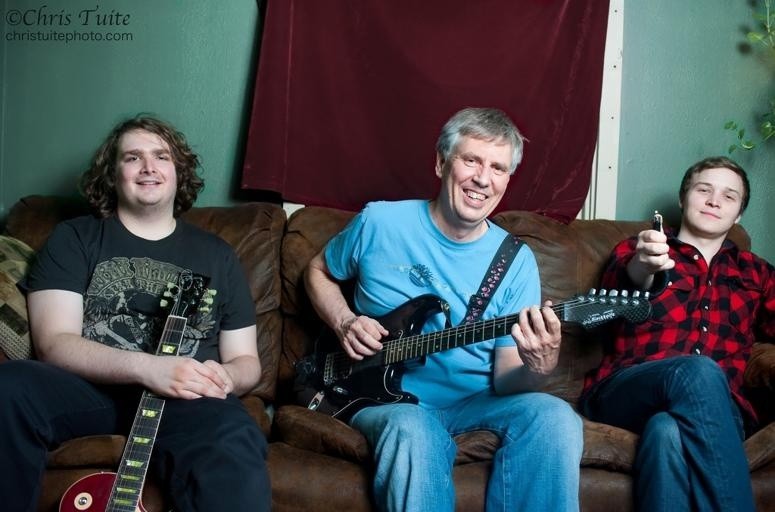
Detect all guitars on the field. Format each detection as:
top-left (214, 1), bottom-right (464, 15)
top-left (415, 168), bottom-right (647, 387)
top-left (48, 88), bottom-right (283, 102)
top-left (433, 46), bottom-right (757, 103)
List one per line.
top-left (60, 272), bottom-right (212, 512)
top-left (292, 288), bottom-right (655, 421)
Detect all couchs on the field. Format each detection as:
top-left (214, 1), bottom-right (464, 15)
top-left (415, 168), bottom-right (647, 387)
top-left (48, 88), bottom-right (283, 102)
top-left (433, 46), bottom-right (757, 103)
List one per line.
top-left (0, 195), bottom-right (774, 511)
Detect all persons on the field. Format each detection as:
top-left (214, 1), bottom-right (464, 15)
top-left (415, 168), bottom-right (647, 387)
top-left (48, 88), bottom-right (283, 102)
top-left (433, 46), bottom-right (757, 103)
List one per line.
top-left (576, 156), bottom-right (775, 512)
top-left (1, 112), bottom-right (273, 512)
top-left (304, 107), bottom-right (584, 512)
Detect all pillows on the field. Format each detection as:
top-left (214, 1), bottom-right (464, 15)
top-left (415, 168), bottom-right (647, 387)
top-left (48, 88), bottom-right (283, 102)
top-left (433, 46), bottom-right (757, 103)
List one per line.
top-left (0, 235), bottom-right (38, 359)
